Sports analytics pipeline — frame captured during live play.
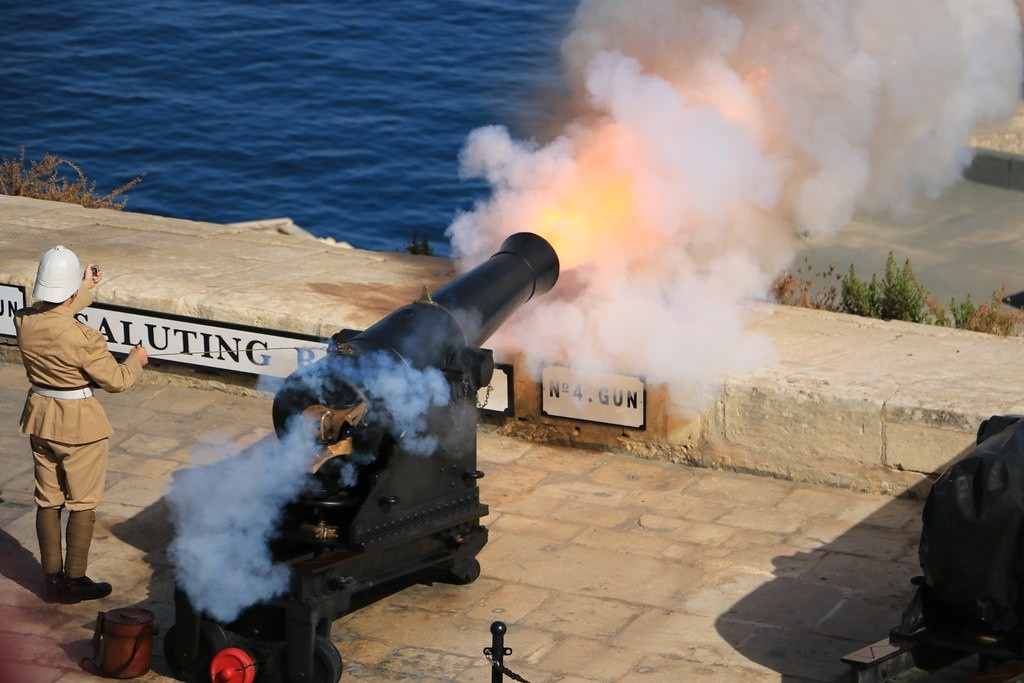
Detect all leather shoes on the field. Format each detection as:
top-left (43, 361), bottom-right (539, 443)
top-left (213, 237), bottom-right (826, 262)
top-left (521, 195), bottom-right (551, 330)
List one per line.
top-left (43, 568), bottom-right (112, 605)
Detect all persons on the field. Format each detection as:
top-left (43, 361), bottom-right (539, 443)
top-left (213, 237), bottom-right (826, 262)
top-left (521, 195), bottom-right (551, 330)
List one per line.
top-left (14, 245), bottom-right (149, 605)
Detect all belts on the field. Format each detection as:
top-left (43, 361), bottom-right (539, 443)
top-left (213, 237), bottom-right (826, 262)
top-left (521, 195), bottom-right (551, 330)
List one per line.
top-left (31, 383), bottom-right (94, 399)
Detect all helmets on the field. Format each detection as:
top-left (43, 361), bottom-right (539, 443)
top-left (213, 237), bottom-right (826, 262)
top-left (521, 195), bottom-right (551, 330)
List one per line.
top-left (32, 245), bottom-right (83, 303)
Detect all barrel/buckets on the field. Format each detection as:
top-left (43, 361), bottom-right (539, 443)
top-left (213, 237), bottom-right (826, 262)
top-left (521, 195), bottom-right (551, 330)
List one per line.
top-left (105, 606), bottom-right (160, 679)
